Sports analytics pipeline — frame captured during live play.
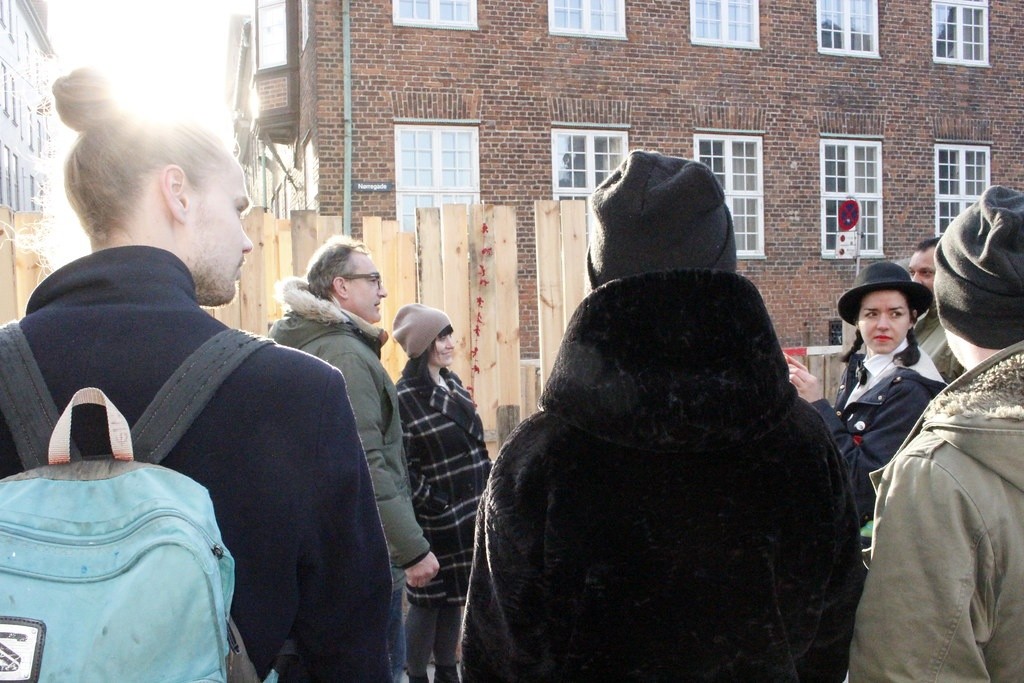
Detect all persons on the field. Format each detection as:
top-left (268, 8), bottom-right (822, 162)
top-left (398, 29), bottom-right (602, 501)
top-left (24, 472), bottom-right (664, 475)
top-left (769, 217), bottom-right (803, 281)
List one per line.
top-left (782, 261), bottom-right (949, 574)
top-left (391, 303), bottom-right (494, 683)
top-left (908, 236), bottom-right (967, 386)
top-left (266, 234), bottom-right (442, 683)
top-left (847, 184), bottom-right (1024, 683)
top-left (0, 78), bottom-right (395, 683)
top-left (458, 148), bottom-right (865, 683)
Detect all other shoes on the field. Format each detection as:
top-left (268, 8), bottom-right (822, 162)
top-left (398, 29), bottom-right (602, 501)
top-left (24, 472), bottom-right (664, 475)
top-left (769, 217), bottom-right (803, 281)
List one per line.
top-left (407, 671), bottom-right (429, 683)
top-left (434, 661), bottom-right (460, 683)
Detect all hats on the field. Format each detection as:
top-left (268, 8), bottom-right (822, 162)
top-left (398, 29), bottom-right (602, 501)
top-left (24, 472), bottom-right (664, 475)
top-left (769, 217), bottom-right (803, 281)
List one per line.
top-left (935, 186), bottom-right (1024, 349)
top-left (393, 303), bottom-right (451, 359)
top-left (581, 150), bottom-right (735, 291)
top-left (836, 263), bottom-right (931, 326)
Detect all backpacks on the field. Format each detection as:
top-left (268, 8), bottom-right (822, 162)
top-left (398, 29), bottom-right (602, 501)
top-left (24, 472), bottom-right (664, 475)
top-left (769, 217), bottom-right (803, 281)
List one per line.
top-left (0, 319), bottom-right (308, 683)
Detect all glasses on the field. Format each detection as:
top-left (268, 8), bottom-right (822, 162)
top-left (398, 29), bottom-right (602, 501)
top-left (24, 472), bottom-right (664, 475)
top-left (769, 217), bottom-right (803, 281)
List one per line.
top-left (341, 272), bottom-right (382, 290)
